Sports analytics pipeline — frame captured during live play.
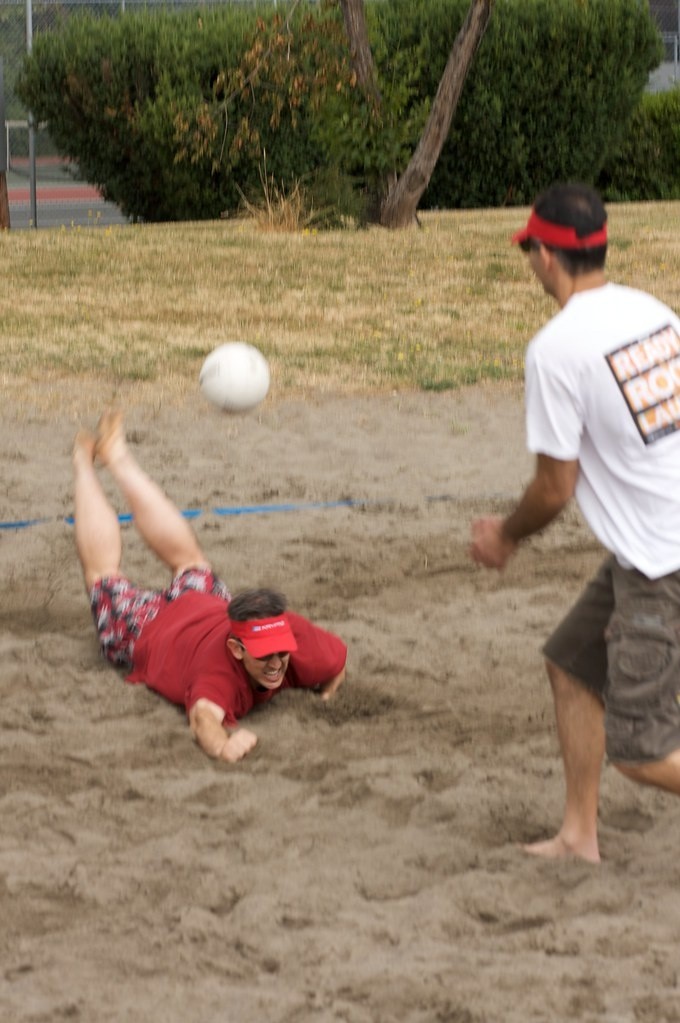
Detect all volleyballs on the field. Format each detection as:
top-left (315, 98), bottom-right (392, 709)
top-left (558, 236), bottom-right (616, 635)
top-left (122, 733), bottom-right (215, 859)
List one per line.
top-left (199, 340), bottom-right (269, 412)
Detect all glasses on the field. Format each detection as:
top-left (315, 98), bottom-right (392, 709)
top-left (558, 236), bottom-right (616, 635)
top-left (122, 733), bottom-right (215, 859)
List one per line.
top-left (237, 640), bottom-right (290, 661)
top-left (519, 238), bottom-right (539, 252)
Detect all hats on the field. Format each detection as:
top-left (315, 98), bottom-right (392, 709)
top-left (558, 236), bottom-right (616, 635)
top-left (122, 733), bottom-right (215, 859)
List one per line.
top-left (228, 613), bottom-right (297, 658)
top-left (511, 203), bottom-right (606, 250)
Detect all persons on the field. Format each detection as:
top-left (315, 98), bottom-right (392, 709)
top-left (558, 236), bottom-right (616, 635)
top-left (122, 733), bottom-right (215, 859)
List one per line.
top-left (466, 184), bottom-right (680, 861)
top-left (72, 412), bottom-right (347, 762)
